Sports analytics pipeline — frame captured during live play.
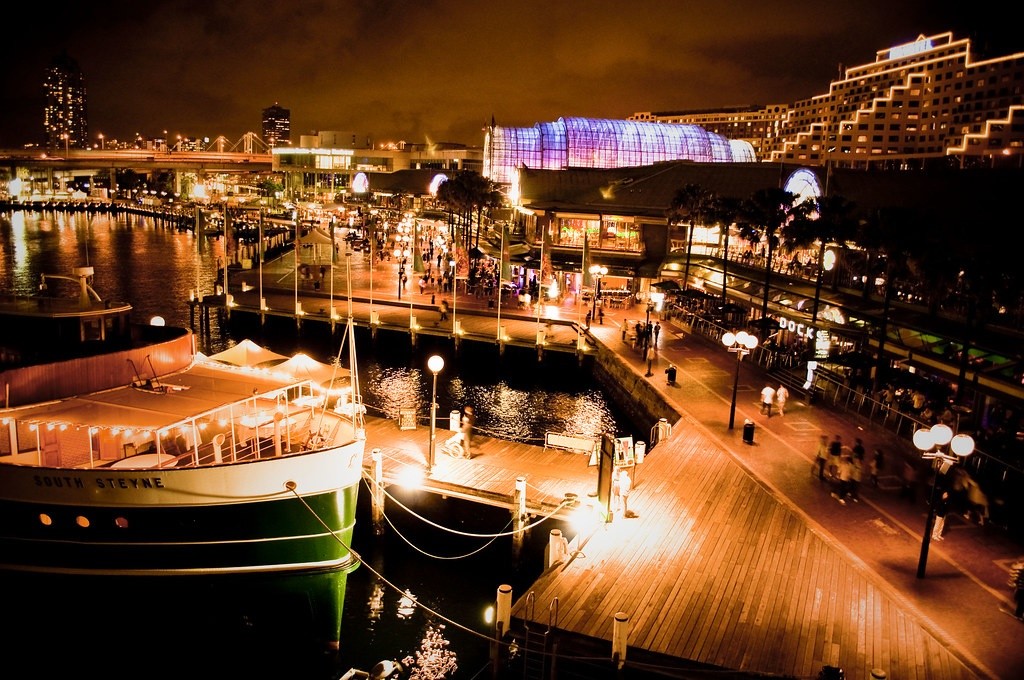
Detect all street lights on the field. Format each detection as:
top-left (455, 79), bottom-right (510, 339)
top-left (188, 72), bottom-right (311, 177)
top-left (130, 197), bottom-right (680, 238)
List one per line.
top-left (911, 424), bottom-right (976, 579)
top-left (64, 134), bottom-right (69, 155)
top-left (636, 290), bottom-right (665, 362)
top-left (588, 265), bottom-right (608, 319)
top-left (427, 355), bottom-right (445, 470)
top-left (98, 134), bottom-right (104, 149)
top-left (721, 331), bottom-right (759, 429)
top-left (396, 213), bottom-right (414, 263)
top-left (394, 250), bottom-right (409, 299)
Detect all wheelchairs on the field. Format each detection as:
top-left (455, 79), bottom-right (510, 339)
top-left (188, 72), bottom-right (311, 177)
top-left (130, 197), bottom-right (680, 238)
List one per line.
top-left (442, 438), bottom-right (465, 459)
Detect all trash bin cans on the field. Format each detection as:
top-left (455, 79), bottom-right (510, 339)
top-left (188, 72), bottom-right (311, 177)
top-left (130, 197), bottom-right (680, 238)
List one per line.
top-left (398, 407), bottom-right (416, 431)
top-left (742, 418), bottom-right (755, 443)
top-left (488, 298), bottom-right (494, 308)
top-left (664, 364), bottom-right (677, 386)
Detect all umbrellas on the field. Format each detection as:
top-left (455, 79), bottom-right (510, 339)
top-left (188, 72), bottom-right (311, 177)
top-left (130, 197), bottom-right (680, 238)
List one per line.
top-left (649, 280), bottom-right (789, 337)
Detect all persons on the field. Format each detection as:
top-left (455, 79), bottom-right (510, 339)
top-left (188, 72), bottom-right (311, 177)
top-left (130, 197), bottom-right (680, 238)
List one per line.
top-left (842, 364), bottom-right (1019, 466)
top-left (422, 238), bottom-right (434, 262)
top-left (1015, 569), bottom-right (1024, 620)
top-left (622, 319), bottom-right (660, 371)
top-left (517, 285), bottom-right (532, 310)
top-left (704, 311), bottom-right (709, 315)
top-left (418, 261), bottom-right (454, 295)
top-left (931, 490), bottom-right (948, 542)
top-left (935, 465), bottom-right (1007, 532)
top-left (620, 471), bottom-right (632, 518)
top-left (401, 272), bottom-right (407, 288)
top-left (811, 434), bottom-right (919, 506)
top-left (437, 253), bottom-right (442, 267)
top-left (586, 310), bottom-right (591, 328)
top-left (439, 300), bottom-right (449, 321)
top-left (353, 231), bottom-right (356, 237)
top-left (762, 338), bottom-right (784, 349)
top-left (700, 302), bottom-right (704, 308)
top-left (760, 383), bottom-right (789, 418)
top-left (461, 406), bottom-right (474, 459)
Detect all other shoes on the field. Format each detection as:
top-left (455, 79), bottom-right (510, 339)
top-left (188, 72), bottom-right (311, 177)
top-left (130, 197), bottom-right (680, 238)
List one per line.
top-left (832, 493), bottom-right (846, 503)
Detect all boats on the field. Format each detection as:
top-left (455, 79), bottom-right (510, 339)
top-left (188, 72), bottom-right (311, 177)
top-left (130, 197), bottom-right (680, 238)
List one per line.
top-left (0, 293), bottom-right (367, 665)
